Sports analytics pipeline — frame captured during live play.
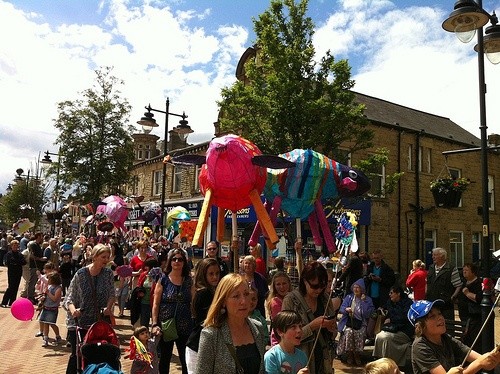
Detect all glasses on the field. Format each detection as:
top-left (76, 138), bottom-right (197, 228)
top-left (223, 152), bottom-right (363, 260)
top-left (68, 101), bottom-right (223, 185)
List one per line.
top-left (391, 292), bottom-right (394, 294)
top-left (151, 242), bottom-right (154, 243)
top-left (55, 242), bottom-right (57, 243)
top-left (208, 248), bottom-right (216, 250)
top-left (307, 280), bottom-right (326, 290)
top-left (171, 257), bottom-right (183, 262)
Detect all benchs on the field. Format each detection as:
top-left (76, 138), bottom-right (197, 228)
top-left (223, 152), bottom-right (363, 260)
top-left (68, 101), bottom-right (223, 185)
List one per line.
top-left (445, 319), bottom-right (473, 342)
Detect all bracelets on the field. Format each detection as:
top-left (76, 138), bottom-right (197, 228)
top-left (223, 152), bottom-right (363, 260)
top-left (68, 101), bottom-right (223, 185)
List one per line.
top-left (153, 323), bottom-right (158, 328)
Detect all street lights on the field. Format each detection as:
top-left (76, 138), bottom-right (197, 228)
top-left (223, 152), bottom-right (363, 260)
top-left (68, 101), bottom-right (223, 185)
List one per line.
top-left (441, 0), bottom-right (500, 374)
top-left (40, 145), bottom-right (72, 237)
top-left (137, 96), bottom-right (195, 241)
top-left (13, 166), bottom-right (41, 220)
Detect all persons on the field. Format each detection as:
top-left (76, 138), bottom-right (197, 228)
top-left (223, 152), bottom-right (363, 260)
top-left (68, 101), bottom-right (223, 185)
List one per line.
top-left (336, 248), bottom-right (500, 374)
top-left (34, 261), bottom-right (62, 347)
top-left (130, 326), bottom-right (162, 374)
top-left (0, 228), bottom-right (75, 308)
top-left (152, 241), bottom-right (338, 374)
top-left (63, 242), bottom-right (115, 374)
top-left (72, 233), bottom-right (157, 330)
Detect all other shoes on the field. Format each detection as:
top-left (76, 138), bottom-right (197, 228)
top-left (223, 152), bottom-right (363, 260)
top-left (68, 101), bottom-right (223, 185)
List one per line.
top-left (0, 304), bottom-right (8, 308)
top-left (347, 359), bottom-right (353, 364)
top-left (355, 360), bottom-right (361, 365)
top-left (119, 314), bottom-right (123, 318)
top-left (54, 343), bottom-right (61, 351)
top-left (28, 298), bottom-right (36, 305)
top-left (42, 343), bottom-right (49, 347)
top-left (35, 332), bottom-right (43, 337)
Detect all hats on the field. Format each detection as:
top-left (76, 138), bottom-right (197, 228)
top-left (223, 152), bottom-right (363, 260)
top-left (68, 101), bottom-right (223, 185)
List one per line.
top-left (64, 238), bottom-right (70, 242)
top-left (483, 278), bottom-right (494, 289)
top-left (407, 300), bottom-right (445, 327)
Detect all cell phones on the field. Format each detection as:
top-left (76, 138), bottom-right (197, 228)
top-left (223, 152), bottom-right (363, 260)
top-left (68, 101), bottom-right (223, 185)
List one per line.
top-left (327, 315), bottom-right (335, 320)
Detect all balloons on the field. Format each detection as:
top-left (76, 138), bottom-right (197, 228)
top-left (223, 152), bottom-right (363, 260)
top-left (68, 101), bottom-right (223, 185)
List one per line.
top-left (11, 298), bottom-right (34, 321)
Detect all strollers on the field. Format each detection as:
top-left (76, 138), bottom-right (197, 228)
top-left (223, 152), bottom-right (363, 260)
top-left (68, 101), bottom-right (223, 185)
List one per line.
top-left (76, 307), bottom-right (124, 374)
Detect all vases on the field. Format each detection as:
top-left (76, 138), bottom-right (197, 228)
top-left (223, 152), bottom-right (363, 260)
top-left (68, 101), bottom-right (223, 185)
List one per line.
top-left (432, 187), bottom-right (464, 209)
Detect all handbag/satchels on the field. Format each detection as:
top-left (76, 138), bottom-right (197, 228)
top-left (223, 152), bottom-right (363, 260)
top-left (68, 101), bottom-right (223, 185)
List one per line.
top-left (384, 324), bottom-right (399, 333)
top-left (161, 319), bottom-right (179, 342)
top-left (45, 262), bottom-right (54, 269)
top-left (346, 317), bottom-right (363, 330)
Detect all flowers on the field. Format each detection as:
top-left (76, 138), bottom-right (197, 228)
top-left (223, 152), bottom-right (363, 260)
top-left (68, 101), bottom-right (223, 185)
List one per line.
top-left (430, 178), bottom-right (470, 192)
